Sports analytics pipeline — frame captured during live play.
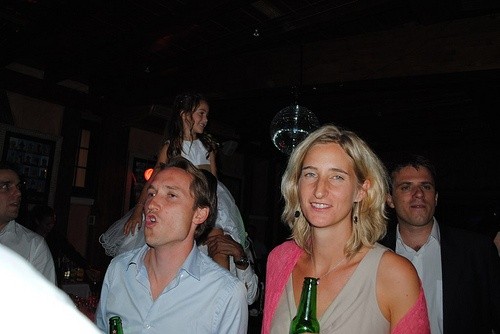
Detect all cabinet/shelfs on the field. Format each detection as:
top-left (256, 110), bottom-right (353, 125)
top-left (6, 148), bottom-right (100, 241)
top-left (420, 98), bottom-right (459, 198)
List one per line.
top-left (123, 152), bottom-right (245, 217)
top-left (1, 123), bottom-right (62, 210)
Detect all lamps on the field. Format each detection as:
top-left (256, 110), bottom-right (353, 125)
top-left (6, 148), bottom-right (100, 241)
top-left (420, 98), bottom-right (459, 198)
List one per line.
top-left (269, 25), bottom-right (320, 154)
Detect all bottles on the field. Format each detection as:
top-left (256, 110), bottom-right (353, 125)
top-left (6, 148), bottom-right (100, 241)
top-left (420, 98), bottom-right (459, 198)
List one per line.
top-left (288, 276), bottom-right (321, 334)
top-left (57, 257), bottom-right (63, 280)
top-left (109, 316), bottom-right (123, 334)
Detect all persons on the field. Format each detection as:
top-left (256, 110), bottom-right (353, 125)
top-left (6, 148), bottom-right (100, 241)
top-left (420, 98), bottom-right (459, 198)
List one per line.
top-left (261, 126), bottom-right (430, 334)
top-left (100, 94), bottom-right (251, 273)
top-left (0, 168), bottom-right (56, 285)
top-left (193, 221), bottom-right (259, 305)
top-left (378, 158), bottom-right (500, 334)
top-left (96, 158), bottom-right (248, 334)
top-left (25, 206), bottom-right (102, 283)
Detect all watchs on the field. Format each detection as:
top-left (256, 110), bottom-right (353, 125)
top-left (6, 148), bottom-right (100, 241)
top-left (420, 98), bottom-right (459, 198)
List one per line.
top-left (234, 255), bottom-right (249, 265)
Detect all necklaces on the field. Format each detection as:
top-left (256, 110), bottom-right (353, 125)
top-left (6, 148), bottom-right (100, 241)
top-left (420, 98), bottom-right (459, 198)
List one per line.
top-left (309, 257), bottom-right (345, 285)
